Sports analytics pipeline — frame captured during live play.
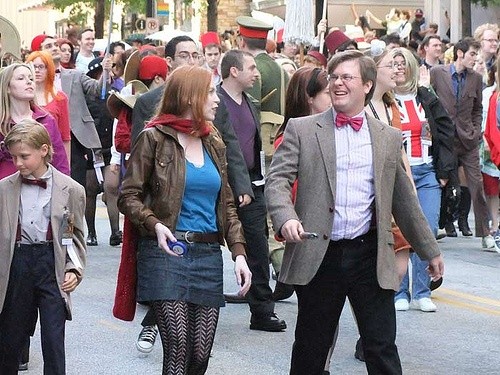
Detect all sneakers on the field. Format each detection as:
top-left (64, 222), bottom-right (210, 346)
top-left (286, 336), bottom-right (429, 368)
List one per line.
top-left (250, 312), bottom-right (287, 330)
top-left (394, 297), bottom-right (436, 312)
top-left (136, 323), bottom-right (159, 354)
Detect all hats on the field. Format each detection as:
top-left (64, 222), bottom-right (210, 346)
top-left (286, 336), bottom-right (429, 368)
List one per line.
top-left (201, 32), bottom-right (219, 47)
top-left (414, 9), bottom-right (423, 15)
top-left (326, 30), bottom-right (355, 55)
top-left (307, 50), bottom-right (327, 67)
top-left (235, 15), bottom-right (274, 39)
top-left (369, 38), bottom-right (386, 56)
top-left (86, 56), bottom-right (116, 77)
top-left (31, 34), bottom-right (53, 53)
top-left (76, 27), bottom-right (94, 38)
top-left (139, 43), bottom-right (158, 56)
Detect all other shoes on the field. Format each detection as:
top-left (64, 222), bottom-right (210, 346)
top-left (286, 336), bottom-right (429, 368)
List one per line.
top-left (223, 290), bottom-right (248, 303)
top-left (272, 272), bottom-right (295, 301)
top-left (481, 234), bottom-right (496, 251)
top-left (87, 234), bottom-right (97, 246)
top-left (110, 231), bottom-right (122, 246)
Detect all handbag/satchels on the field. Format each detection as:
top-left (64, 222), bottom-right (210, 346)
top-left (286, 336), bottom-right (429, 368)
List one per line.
top-left (441, 183), bottom-right (461, 222)
top-left (259, 110), bottom-right (285, 160)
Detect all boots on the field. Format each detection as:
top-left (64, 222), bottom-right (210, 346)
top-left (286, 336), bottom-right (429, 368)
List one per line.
top-left (458, 186), bottom-right (478, 242)
top-left (445, 222), bottom-right (457, 237)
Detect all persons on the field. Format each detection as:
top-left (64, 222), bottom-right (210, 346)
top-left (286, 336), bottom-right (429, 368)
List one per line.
top-left (1, 3), bottom-right (500, 375)
top-left (265, 50), bottom-right (446, 375)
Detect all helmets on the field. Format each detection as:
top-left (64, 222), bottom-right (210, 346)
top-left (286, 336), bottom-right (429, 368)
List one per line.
top-left (137, 55), bottom-right (170, 82)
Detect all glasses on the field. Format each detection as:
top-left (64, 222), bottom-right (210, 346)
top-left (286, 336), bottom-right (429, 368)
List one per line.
top-left (377, 61), bottom-right (395, 69)
top-left (393, 61), bottom-right (410, 68)
top-left (482, 39), bottom-right (498, 42)
top-left (326, 73), bottom-right (361, 82)
top-left (34, 65), bottom-right (47, 71)
top-left (174, 52), bottom-right (199, 60)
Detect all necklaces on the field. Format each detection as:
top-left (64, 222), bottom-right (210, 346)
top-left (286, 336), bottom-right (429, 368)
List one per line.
top-left (39, 91), bottom-right (50, 106)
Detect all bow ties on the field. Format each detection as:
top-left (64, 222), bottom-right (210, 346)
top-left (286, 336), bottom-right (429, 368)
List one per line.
top-left (337, 111), bottom-right (364, 131)
top-left (22, 178), bottom-right (46, 189)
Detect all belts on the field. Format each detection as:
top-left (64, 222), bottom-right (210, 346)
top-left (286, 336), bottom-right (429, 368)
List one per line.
top-left (173, 230), bottom-right (220, 243)
